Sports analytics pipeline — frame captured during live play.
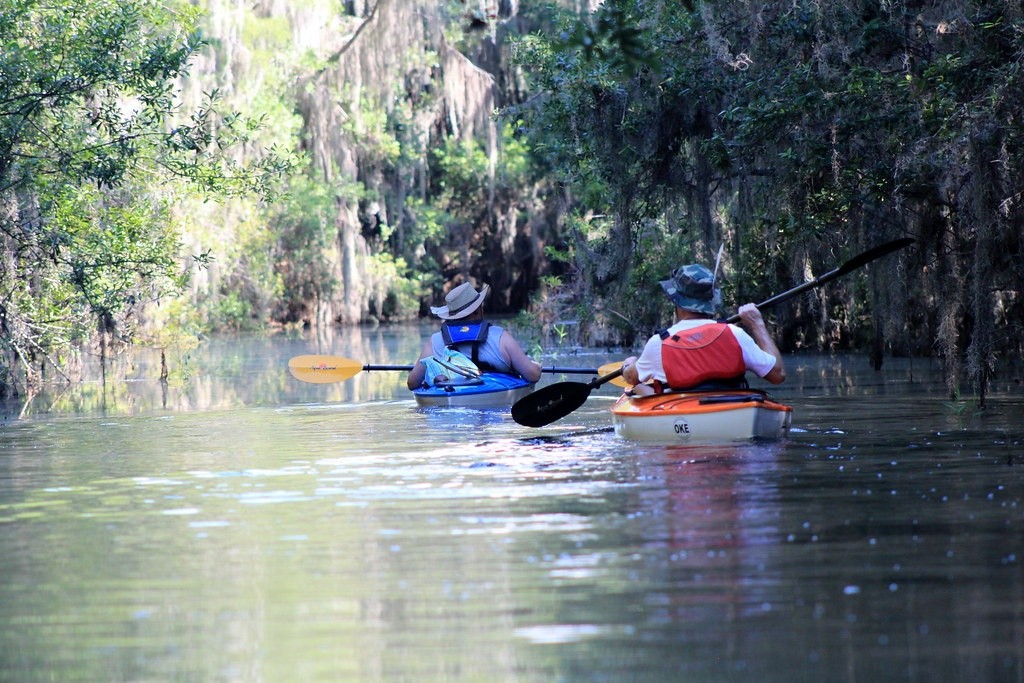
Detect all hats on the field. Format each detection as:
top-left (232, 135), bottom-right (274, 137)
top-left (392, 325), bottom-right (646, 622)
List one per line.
top-left (657, 264), bottom-right (721, 314)
top-left (430, 282), bottom-right (489, 320)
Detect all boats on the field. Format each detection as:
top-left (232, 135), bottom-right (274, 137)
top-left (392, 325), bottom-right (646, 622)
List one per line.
top-left (413, 372), bottom-right (537, 412)
top-left (612, 386), bottom-right (794, 448)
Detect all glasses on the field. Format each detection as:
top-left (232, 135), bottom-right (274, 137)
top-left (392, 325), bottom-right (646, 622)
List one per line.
top-left (671, 268), bottom-right (684, 291)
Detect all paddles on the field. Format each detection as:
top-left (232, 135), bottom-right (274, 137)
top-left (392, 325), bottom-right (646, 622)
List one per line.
top-left (285, 351), bottom-right (641, 390)
top-left (511, 230), bottom-right (920, 432)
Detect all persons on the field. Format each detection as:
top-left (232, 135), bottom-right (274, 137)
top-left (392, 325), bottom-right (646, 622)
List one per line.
top-left (407, 282), bottom-right (542, 391)
top-left (621, 263), bottom-right (786, 396)
